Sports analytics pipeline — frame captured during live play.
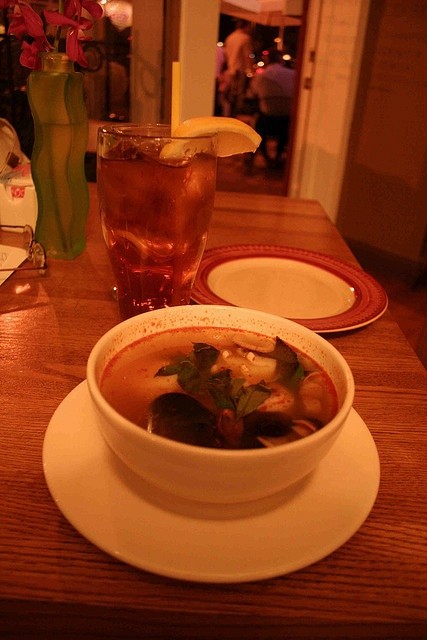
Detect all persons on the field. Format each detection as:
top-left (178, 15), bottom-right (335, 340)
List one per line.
top-left (224, 18), bottom-right (256, 121)
top-left (251, 50), bottom-right (297, 176)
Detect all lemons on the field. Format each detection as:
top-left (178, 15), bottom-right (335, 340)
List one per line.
top-left (155, 116), bottom-right (261, 162)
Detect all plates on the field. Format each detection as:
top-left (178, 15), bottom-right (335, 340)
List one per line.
top-left (190, 245), bottom-right (389, 332)
top-left (0, 118), bottom-right (38, 285)
top-left (42, 378), bottom-right (380, 583)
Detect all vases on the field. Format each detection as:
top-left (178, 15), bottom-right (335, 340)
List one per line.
top-left (29, 70), bottom-right (93, 261)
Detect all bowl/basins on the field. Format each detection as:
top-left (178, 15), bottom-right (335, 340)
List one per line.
top-left (87, 305), bottom-right (356, 501)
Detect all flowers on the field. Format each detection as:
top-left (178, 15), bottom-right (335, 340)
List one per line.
top-left (6, 0), bottom-right (106, 75)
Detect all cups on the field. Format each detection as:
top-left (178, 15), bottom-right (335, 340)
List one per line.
top-left (97, 123), bottom-right (218, 320)
